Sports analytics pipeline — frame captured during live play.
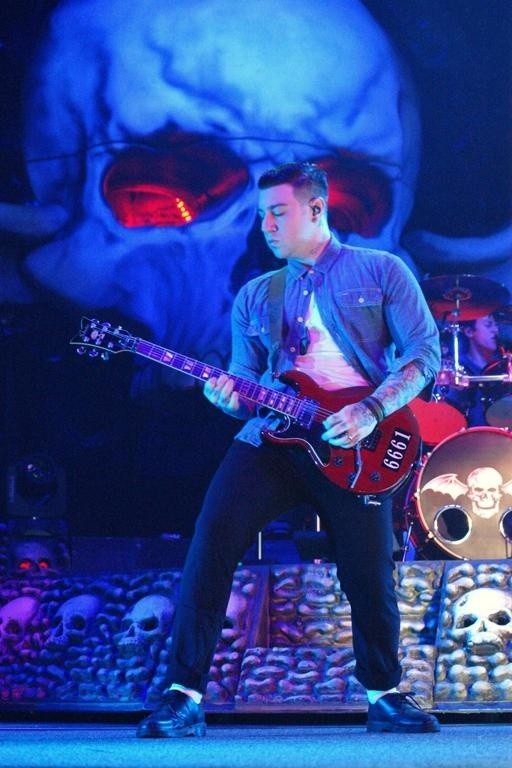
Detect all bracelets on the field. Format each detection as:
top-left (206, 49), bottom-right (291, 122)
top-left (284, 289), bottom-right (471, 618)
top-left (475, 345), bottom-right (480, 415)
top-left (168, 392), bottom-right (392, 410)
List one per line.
top-left (362, 397), bottom-right (386, 424)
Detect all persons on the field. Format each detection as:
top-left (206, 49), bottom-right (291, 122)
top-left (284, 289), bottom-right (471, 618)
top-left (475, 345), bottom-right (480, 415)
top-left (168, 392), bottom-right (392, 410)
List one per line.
top-left (452, 313), bottom-right (512, 428)
top-left (138, 162), bottom-right (442, 738)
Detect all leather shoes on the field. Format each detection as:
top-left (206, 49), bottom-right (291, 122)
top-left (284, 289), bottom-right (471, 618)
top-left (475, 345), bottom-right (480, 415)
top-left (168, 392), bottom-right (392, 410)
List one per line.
top-left (367, 690), bottom-right (439, 733)
top-left (137, 689), bottom-right (207, 739)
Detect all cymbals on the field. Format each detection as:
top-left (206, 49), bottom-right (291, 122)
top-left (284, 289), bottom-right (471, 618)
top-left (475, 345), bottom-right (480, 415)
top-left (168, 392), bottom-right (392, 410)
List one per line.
top-left (417, 274), bottom-right (507, 323)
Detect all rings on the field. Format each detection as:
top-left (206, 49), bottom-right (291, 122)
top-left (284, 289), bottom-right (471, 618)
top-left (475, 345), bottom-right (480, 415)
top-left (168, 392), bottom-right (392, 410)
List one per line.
top-left (347, 435), bottom-right (353, 441)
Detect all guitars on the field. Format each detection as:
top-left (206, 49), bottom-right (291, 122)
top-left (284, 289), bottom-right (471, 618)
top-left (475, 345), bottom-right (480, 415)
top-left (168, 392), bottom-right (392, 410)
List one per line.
top-left (70, 317), bottom-right (426, 495)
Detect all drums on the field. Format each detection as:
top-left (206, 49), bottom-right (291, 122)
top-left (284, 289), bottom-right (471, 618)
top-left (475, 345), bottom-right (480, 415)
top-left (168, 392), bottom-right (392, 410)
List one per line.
top-left (407, 357), bottom-right (477, 444)
top-left (480, 353), bottom-right (512, 427)
top-left (406, 425), bottom-right (511, 560)
